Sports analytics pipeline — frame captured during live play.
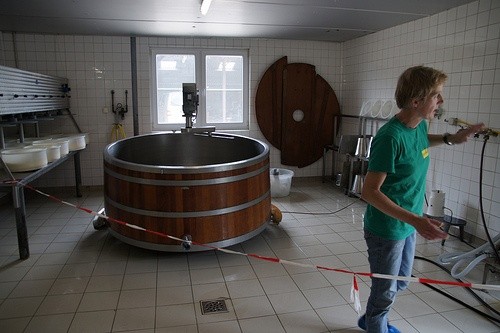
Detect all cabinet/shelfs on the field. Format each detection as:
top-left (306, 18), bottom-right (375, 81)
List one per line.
top-left (323, 112), bottom-right (392, 204)
top-left (0, 66), bottom-right (88, 260)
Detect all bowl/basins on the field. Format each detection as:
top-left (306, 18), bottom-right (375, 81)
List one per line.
top-left (2, 132), bottom-right (89, 172)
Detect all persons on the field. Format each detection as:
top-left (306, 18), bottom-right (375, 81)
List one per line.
top-left (358, 65), bottom-right (486, 333)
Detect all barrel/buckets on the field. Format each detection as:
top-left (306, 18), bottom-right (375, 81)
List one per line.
top-left (270, 168), bottom-right (294, 197)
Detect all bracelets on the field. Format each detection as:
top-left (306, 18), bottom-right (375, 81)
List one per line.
top-left (442, 133), bottom-right (452, 145)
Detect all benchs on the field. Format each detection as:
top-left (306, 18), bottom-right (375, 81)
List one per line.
top-left (423, 210), bottom-right (467, 247)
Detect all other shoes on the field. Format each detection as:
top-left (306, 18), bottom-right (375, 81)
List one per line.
top-left (358, 314), bottom-right (400, 333)
